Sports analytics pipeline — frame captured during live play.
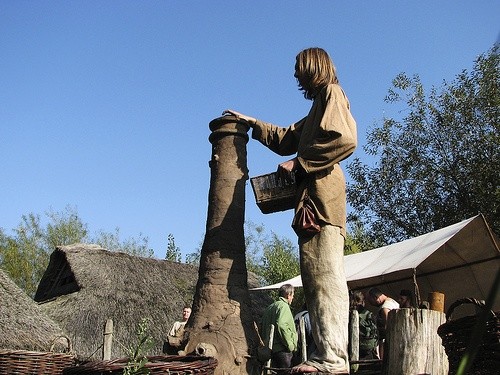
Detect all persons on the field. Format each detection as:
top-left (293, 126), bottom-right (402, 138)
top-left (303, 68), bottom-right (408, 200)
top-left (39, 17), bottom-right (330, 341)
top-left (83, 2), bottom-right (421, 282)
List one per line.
top-left (261, 284), bottom-right (298, 375)
top-left (295, 303), bottom-right (316, 361)
top-left (167, 306), bottom-right (192, 355)
top-left (348, 291), bottom-right (379, 360)
top-left (222, 48), bottom-right (358, 374)
top-left (398, 290), bottom-right (414, 308)
top-left (370, 288), bottom-right (400, 343)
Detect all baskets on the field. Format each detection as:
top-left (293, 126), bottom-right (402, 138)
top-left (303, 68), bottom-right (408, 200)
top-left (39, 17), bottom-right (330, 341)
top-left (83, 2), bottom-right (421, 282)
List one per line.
top-left (436, 295), bottom-right (499, 375)
top-left (167, 327), bottom-right (182, 347)
top-left (250, 168), bottom-right (304, 215)
top-left (61, 354), bottom-right (219, 375)
top-left (0, 334), bottom-right (77, 375)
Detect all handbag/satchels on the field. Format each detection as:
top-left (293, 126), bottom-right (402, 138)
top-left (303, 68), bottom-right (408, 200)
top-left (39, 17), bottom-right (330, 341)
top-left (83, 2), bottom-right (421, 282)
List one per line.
top-left (291, 194), bottom-right (321, 239)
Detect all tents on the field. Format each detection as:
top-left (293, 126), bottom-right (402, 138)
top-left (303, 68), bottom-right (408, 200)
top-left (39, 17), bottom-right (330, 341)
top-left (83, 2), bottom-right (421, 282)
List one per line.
top-left (248, 213), bottom-right (500, 323)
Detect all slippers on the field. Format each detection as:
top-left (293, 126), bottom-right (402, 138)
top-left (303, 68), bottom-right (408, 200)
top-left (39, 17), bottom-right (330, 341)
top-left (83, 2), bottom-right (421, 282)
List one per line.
top-left (295, 359), bottom-right (350, 375)
